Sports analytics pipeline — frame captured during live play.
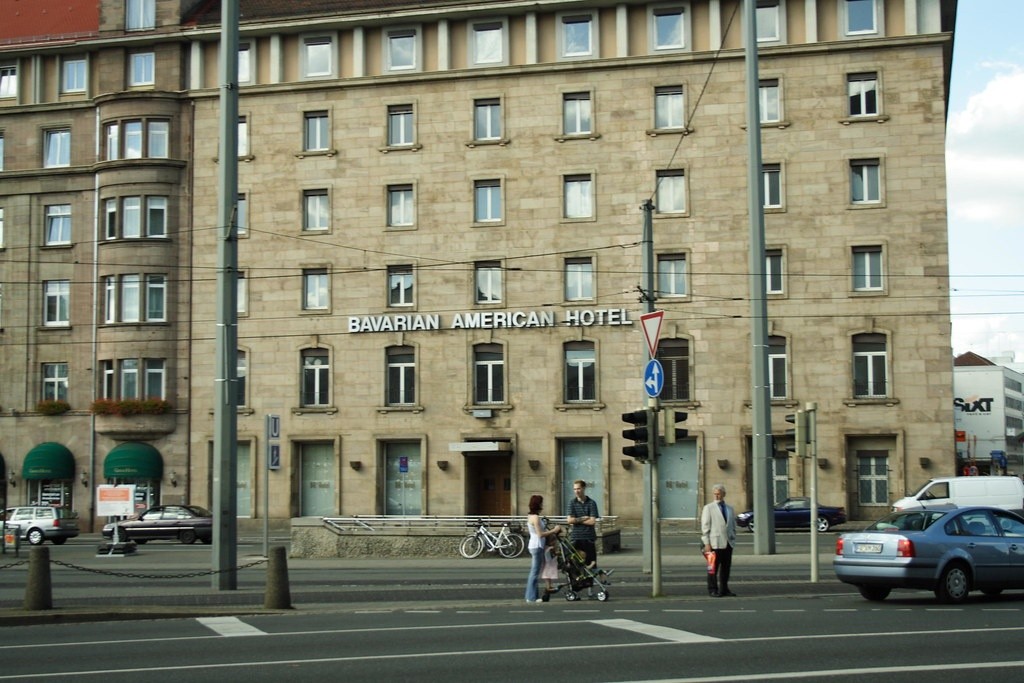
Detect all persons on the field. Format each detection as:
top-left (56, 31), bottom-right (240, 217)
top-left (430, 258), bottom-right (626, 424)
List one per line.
top-left (566, 480), bottom-right (600, 599)
top-left (524, 494), bottom-right (558, 604)
top-left (700, 485), bottom-right (736, 597)
top-left (577, 548), bottom-right (612, 585)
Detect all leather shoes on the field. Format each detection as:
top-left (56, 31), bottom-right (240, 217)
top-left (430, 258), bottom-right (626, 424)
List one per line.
top-left (710, 591), bottom-right (721, 596)
top-left (721, 591), bottom-right (736, 596)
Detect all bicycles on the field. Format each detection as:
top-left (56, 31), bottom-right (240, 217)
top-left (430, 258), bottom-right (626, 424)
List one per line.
top-left (459, 517), bottom-right (525, 559)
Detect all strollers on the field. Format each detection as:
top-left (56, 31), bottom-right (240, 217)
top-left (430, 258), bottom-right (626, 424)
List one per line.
top-left (544, 524), bottom-right (609, 602)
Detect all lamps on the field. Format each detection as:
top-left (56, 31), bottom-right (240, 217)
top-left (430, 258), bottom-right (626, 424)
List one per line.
top-left (817, 458), bottom-right (827, 469)
top-left (8, 469), bottom-right (17, 488)
top-left (528, 460), bottom-right (540, 470)
top-left (717, 458), bottom-right (728, 469)
top-left (920, 457), bottom-right (930, 468)
top-left (622, 459), bottom-right (632, 470)
top-left (437, 461), bottom-right (449, 471)
top-left (350, 460), bottom-right (362, 469)
top-left (81, 469), bottom-right (89, 486)
top-left (169, 471), bottom-right (178, 486)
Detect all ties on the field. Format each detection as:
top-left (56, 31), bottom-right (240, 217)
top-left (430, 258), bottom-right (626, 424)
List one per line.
top-left (721, 505), bottom-right (726, 522)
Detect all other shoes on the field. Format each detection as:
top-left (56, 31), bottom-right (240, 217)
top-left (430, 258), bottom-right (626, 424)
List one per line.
top-left (588, 593), bottom-right (598, 600)
top-left (526, 598), bottom-right (543, 602)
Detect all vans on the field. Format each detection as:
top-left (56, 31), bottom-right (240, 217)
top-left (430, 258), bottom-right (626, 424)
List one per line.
top-left (890, 475), bottom-right (1024, 536)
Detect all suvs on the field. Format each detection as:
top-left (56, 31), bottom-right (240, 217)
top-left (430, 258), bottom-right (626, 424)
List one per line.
top-left (0, 507), bottom-right (80, 545)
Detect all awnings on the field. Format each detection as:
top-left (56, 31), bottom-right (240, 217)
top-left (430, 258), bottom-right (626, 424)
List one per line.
top-left (18, 441), bottom-right (163, 480)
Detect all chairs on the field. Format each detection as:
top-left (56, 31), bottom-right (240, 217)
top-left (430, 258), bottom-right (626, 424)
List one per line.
top-left (969, 522), bottom-right (985, 535)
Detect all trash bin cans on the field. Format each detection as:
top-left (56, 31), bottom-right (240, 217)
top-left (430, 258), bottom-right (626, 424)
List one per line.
top-left (4, 524), bottom-right (21, 552)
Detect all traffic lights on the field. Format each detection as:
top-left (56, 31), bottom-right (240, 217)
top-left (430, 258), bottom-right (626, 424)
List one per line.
top-left (621, 408), bottom-right (656, 461)
top-left (784, 410), bottom-right (808, 459)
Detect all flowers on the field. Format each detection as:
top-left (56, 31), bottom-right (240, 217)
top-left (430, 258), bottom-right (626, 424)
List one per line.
top-left (90, 397), bottom-right (176, 418)
top-left (39, 401), bottom-right (71, 416)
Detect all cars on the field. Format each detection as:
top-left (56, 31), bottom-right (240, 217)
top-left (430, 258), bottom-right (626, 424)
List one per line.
top-left (102, 504), bottom-right (212, 545)
top-left (832, 503), bottom-right (1024, 604)
top-left (735, 496), bottom-right (846, 532)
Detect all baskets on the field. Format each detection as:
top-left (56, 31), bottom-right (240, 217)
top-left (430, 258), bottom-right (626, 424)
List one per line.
top-left (510, 525), bottom-right (521, 532)
